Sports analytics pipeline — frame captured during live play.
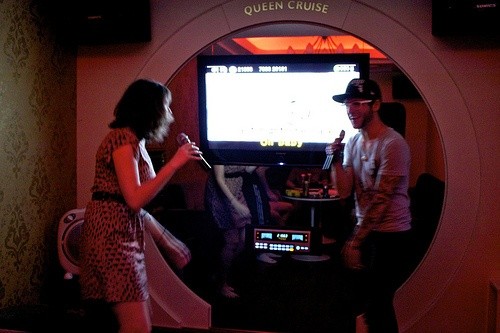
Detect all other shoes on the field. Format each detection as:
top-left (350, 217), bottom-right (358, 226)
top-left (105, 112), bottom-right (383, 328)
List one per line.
top-left (258, 251), bottom-right (277, 264)
top-left (221, 289), bottom-right (241, 299)
top-left (263, 251), bottom-right (282, 258)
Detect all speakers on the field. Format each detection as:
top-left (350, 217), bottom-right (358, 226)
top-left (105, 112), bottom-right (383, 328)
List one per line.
top-left (46, 0), bottom-right (151, 44)
top-left (431, 0), bottom-right (500, 47)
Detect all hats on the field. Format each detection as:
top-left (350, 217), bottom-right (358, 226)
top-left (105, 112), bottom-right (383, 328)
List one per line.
top-left (332, 78), bottom-right (382, 103)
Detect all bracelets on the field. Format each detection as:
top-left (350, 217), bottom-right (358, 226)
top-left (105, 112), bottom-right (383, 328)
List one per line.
top-left (346, 240), bottom-right (361, 250)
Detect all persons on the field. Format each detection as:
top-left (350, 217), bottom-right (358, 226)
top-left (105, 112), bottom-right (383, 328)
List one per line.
top-left (206, 164), bottom-right (297, 297)
top-left (326, 79), bottom-right (413, 329)
top-left (80, 80), bottom-right (202, 333)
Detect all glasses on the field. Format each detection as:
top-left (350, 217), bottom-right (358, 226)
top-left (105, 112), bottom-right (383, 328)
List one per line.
top-left (341, 98), bottom-right (375, 107)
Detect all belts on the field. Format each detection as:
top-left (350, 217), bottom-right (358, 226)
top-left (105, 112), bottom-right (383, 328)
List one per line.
top-left (225, 172), bottom-right (245, 177)
top-left (91, 191), bottom-right (128, 204)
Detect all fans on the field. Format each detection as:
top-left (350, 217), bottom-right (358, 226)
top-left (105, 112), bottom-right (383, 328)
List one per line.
top-left (58, 209), bottom-right (86, 279)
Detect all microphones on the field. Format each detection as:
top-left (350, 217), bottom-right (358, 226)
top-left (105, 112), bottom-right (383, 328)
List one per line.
top-left (176, 132), bottom-right (212, 172)
top-left (322, 129), bottom-right (345, 171)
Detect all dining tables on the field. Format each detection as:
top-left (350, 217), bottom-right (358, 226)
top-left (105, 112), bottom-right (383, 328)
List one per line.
top-left (283, 194), bottom-right (343, 262)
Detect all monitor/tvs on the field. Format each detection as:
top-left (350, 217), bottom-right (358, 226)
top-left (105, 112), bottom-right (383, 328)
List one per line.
top-left (196, 52), bottom-right (369, 167)
